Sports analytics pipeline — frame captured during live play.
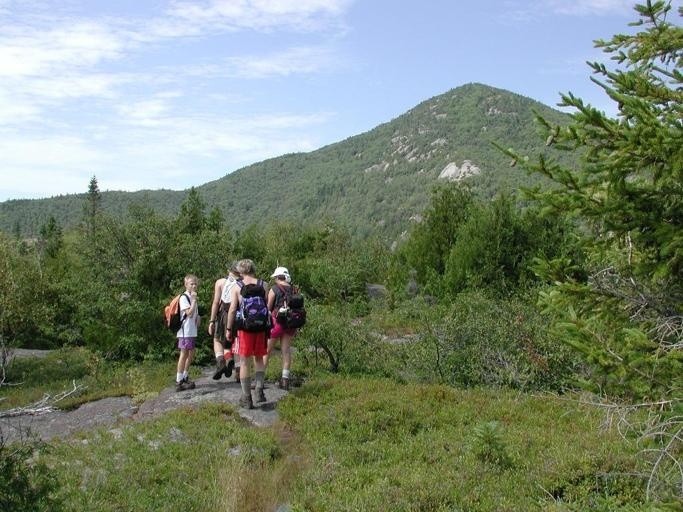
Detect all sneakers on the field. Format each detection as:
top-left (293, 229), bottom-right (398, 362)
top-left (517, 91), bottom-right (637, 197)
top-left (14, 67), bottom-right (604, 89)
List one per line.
top-left (176, 378), bottom-right (196, 391)
top-left (253, 387), bottom-right (266, 402)
top-left (213, 358), bottom-right (242, 383)
top-left (239, 394), bottom-right (253, 409)
top-left (278, 378), bottom-right (290, 390)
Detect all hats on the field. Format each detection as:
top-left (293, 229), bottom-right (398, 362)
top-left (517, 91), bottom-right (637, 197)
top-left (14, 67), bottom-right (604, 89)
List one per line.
top-left (270, 267), bottom-right (292, 282)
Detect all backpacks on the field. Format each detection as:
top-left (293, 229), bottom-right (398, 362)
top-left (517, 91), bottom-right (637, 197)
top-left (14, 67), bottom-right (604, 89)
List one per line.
top-left (235, 279), bottom-right (271, 334)
top-left (163, 294), bottom-right (190, 333)
top-left (275, 283), bottom-right (306, 330)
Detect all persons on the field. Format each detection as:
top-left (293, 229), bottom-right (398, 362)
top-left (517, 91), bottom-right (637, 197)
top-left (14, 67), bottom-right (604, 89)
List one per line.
top-left (209, 258), bottom-right (306, 410)
top-left (165, 275), bottom-right (202, 392)
